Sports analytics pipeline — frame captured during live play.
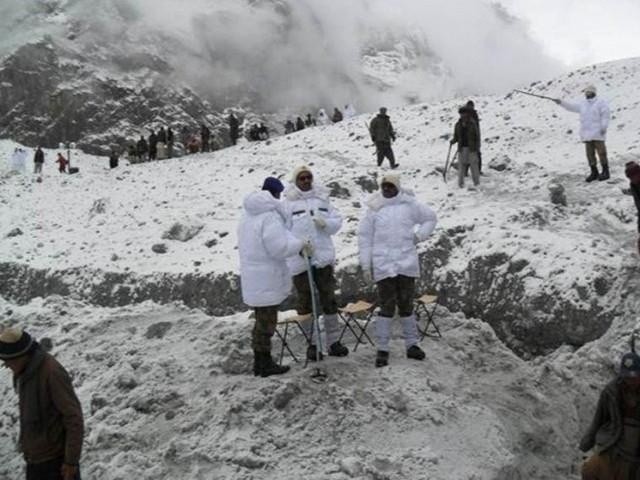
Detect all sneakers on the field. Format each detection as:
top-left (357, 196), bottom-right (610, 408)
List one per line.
top-left (307, 345), bottom-right (323, 361)
top-left (407, 345), bottom-right (425, 360)
top-left (328, 341), bottom-right (348, 357)
top-left (254, 365), bottom-right (290, 377)
top-left (375, 351), bottom-right (389, 367)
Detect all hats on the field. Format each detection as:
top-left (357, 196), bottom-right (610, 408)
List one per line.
top-left (619, 353), bottom-right (640, 380)
top-left (625, 162), bottom-right (640, 177)
top-left (293, 165), bottom-right (313, 181)
top-left (1, 326), bottom-right (33, 358)
top-left (586, 85), bottom-right (596, 95)
top-left (381, 172), bottom-right (401, 192)
top-left (263, 178), bottom-right (284, 192)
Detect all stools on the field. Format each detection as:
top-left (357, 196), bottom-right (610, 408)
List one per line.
top-left (275, 314), bottom-right (316, 368)
top-left (415, 295), bottom-right (441, 340)
top-left (336, 301), bottom-right (378, 352)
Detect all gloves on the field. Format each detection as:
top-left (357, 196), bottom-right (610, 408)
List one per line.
top-left (363, 270), bottom-right (374, 283)
top-left (313, 217), bottom-right (326, 230)
top-left (302, 238), bottom-right (315, 257)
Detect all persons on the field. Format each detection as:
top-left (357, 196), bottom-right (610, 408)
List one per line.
top-left (359, 170), bottom-right (438, 368)
top-left (284, 168), bottom-right (349, 358)
top-left (53, 106), bottom-right (349, 175)
top-left (368, 108), bottom-right (399, 168)
top-left (0, 326), bottom-right (84, 480)
top-left (624, 161), bottom-right (639, 215)
top-left (456, 99), bottom-right (481, 176)
top-left (577, 85), bottom-right (611, 181)
top-left (238, 177), bottom-right (305, 377)
top-left (449, 108), bottom-right (481, 187)
top-left (33, 145), bottom-right (44, 172)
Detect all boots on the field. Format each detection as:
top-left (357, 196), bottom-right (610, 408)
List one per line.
top-left (585, 167), bottom-right (598, 182)
top-left (599, 165), bottom-right (610, 181)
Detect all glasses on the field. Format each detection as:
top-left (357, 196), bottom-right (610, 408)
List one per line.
top-left (298, 176), bottom-right (312, 181)
